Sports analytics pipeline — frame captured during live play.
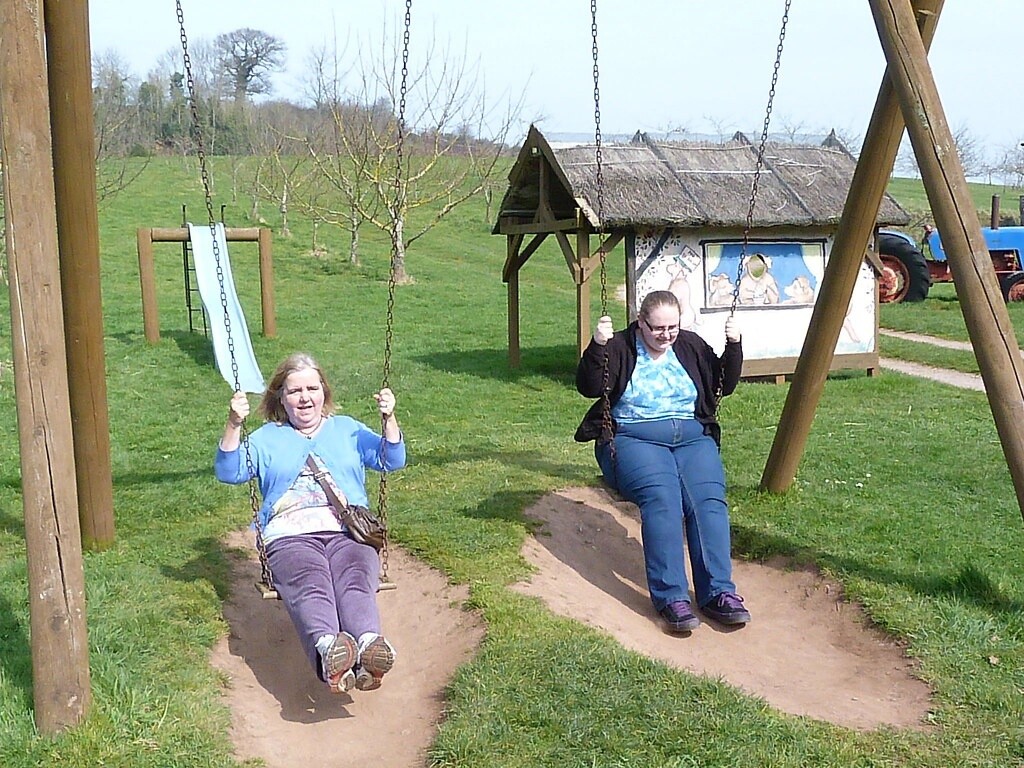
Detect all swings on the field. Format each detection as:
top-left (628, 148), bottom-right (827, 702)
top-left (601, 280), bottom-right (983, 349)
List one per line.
top-left (583, 0), bottom-right (796, 505)
top-left (171, 0), bottom-right (415, 598)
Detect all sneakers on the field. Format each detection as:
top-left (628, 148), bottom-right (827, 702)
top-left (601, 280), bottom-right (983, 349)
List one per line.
top-left (662, 600), bottom-right (698, 631)
top-left (702, 592), bottom-right (751, 624)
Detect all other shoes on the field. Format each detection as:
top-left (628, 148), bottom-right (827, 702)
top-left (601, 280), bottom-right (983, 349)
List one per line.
top-left (324, 631), bottom-right (359, 695)
top-left (355, 635), bottom-right (394, 691)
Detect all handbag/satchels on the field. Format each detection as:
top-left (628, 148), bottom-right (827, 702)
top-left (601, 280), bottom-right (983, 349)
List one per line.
top-left (343, 504), bottom-right (384, 549)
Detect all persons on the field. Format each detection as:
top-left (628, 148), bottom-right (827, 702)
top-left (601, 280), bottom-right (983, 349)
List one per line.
top-left (214, 355), bottom-right (405, 693)
top-left (573, 291), bottom-right (752, 629)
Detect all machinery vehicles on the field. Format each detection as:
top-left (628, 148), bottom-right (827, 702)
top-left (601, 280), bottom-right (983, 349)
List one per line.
top-left (879, 193), bottom-right (1024, 304)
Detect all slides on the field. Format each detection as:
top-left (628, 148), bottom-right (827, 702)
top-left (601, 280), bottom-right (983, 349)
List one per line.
top-left (181, 221), bottom-right (267, 394)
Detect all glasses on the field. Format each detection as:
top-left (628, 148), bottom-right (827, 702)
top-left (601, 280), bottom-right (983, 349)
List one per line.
top-left (643, 318), bottom-right (679, 335)
